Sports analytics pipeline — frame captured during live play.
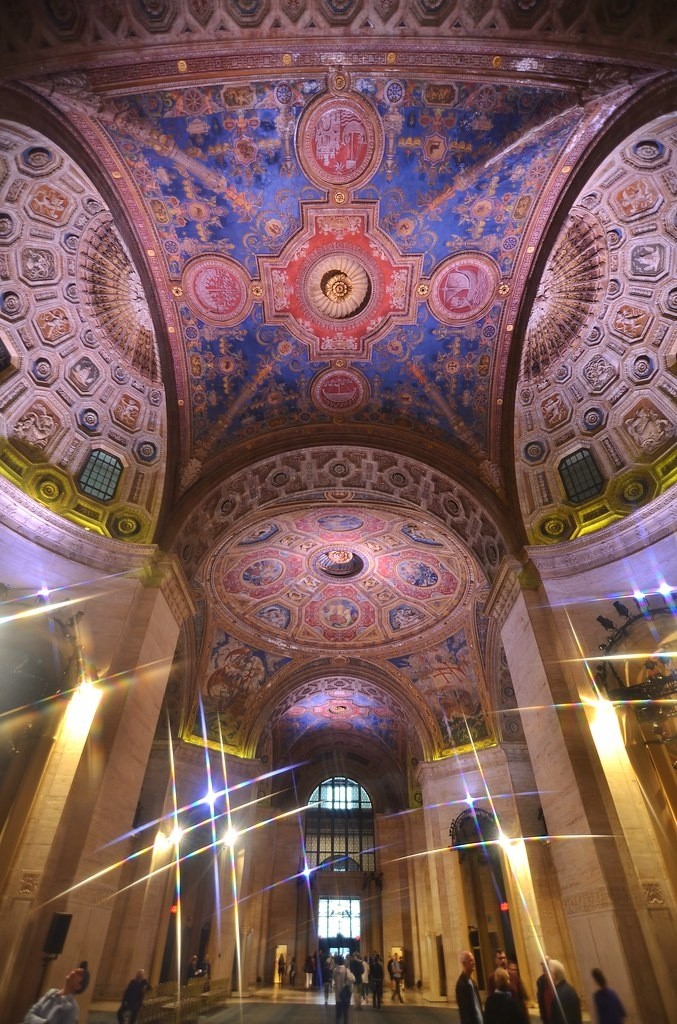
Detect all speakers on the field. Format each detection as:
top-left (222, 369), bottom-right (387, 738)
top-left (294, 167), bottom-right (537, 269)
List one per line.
top-left (44, 912), bottom-right (72, 953)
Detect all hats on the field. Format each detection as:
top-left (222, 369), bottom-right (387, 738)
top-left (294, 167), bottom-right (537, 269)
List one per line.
top-left (76, 960), bottom-right (90, 995)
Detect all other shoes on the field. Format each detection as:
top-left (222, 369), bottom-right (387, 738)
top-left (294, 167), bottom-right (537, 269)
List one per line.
top-left (400, 998), bottom-right (404, 1003)
top-left (391, 994), bottom-right (395, 1001)
top-left (288, 985), bottom-right (295, 990)
top-left (373, 1006), bottom-right (382, 1012)
top-left (353, 1006), bottom-right (363, 1011)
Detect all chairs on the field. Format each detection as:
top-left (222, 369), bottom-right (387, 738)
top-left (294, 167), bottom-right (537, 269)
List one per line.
top-left (135, 974), bottom-right (231, 1024)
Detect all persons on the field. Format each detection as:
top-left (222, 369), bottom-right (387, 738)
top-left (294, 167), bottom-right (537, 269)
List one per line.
top-left (591, 967), bottom-right (629, 1024)
top-left (278, 950), bottom-right (411, 1024)
top-left (183, 953), bottom-right (211, 992)
top-left (484, 950), bottom-right (583, 1024)
top-left (455, 951), bottom-right (485, 1024)
top-left (117, 969), bottom-right (153, 1024)
top-left (23, 959), bottom-right (91, 1024)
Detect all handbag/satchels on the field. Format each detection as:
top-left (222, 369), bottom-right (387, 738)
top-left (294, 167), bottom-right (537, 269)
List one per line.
top-left (387, 958), bottom-right (396, 981)
top-left (339, 985), bottom-right (351, 1003)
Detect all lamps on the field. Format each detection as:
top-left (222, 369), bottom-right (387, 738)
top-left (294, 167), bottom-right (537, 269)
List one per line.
top-left (597, 615), bottom-right (616, 632)
top-left (65, 610), bottom-right (85, 630)
top-left (500, 901), bottom-right (509, 911)
top-left (613, 600), bottom-right (631, 620)
top-left (171, 904), bottom-right (178, 914)
top-left (33, 590), bottom-right (50, 609)
